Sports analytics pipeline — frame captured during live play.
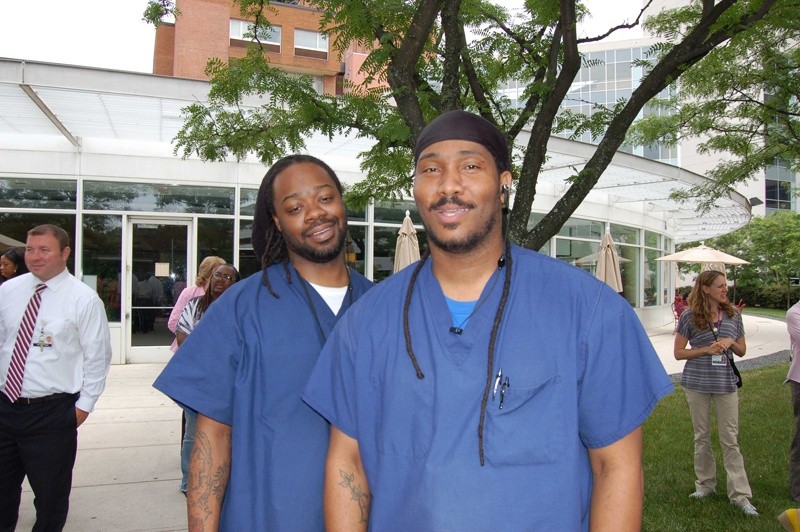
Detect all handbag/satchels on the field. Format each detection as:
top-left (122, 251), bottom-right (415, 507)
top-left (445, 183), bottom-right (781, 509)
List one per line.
top-left (729, 359), bottom-right (742, 389)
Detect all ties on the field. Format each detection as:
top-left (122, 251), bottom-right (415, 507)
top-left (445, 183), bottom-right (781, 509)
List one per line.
top-left (4, 284), bottom-right (48, 403)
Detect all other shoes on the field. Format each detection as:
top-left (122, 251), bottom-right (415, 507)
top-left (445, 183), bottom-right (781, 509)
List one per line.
top-left (689, 488), bottom-right (715, 498)
top-left (737, 498), bottom-right (759, 515)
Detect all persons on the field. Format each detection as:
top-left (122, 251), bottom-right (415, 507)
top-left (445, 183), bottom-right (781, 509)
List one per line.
top-left (782, 299), bottom-right (800, 504)
top-left (152, 154), bottom-right (375, 532)
top-left (0, 247), bottom-right (30, 286)
top-left (0, 224), bottom-right (112, 532)
top-left (675, 288), bottom-right (693, 320)
top-left (675, 270), bottom-right (761, 518)
top-left (166, 255), bottom-right (239, 497)
top-left (300, 109), bottom-right (675, 532)
top-left (97, 266), bottom-right (186, 334)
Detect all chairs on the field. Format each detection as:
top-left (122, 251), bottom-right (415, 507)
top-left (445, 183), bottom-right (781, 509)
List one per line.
top-left (736, 299), bottom-right (745, 313)
top-left (671, 302), bottom-right (680, 336)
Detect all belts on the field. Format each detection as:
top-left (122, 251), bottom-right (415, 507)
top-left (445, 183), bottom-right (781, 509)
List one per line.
top-left (0, 393), bottom-right (72, 404)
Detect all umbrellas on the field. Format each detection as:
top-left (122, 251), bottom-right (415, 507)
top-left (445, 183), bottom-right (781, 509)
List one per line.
top-left (654, 245), bottom-right (751, 271)
top-left (393, 209), bottom-right (420, 275)
top-left (568, 251), bottom-right (632, 266)
top-left (594, 226), bottom-right (624, 293)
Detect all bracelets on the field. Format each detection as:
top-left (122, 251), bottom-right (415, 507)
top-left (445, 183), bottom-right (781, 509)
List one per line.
top-left (704, 346), bottom-right (708, 356)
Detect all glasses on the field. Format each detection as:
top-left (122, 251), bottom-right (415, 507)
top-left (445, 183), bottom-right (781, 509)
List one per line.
top-left (211, 272), bottom-right (238, 282)
